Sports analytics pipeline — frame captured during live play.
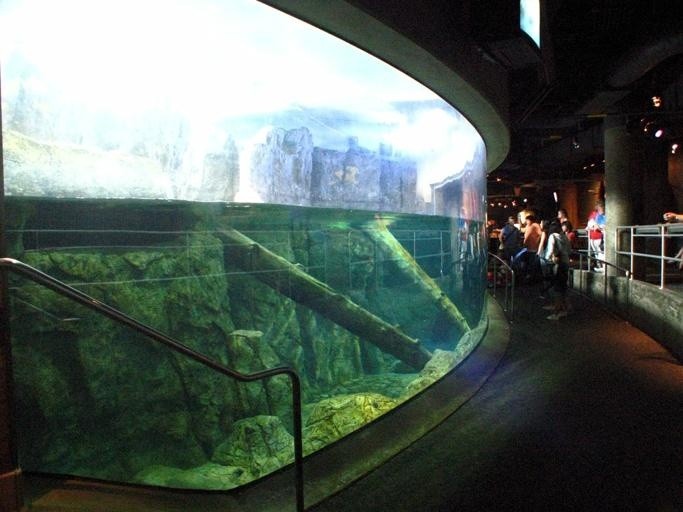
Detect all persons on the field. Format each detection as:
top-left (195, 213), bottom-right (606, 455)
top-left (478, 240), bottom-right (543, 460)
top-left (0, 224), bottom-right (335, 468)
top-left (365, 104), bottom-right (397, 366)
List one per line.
top-left (484, 198), bottom-right (605, 322)
top-left (663, 212), bottom-right (682, 271)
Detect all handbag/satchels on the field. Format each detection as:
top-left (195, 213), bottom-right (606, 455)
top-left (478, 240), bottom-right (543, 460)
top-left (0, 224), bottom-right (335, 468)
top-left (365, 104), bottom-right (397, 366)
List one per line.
top-left (553, 238), bottom-right (569, 257)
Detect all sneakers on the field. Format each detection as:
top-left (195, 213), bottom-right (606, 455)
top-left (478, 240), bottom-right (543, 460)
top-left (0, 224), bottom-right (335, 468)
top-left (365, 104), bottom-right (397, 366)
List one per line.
top-left (539, 295), bottom-right (573, 320)
